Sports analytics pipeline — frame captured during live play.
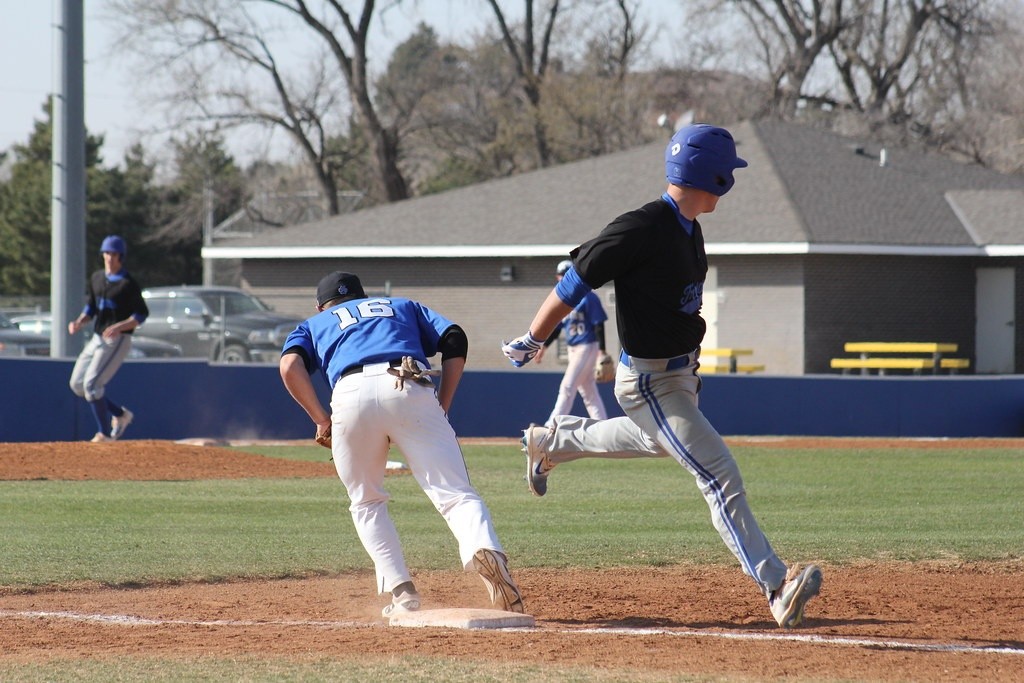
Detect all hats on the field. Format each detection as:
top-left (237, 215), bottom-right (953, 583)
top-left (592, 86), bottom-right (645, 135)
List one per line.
top-left (316, 271), bottom-right (365, 307)
top-left (557, 260), bottom-right (573, 273)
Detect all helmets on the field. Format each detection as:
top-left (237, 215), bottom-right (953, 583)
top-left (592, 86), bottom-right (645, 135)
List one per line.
top-left (665, 123), bottom-right (748, 197)
top-left (100, 235), bottom-right (126, 262)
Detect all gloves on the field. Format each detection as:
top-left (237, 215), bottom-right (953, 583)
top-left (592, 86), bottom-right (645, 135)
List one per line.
top-left (387, 356), bottom-right (441, 391)
top-left (501, 331), bottom-right (546, 368)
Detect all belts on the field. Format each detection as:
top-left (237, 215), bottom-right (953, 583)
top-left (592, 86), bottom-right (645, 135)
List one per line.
top-left (341, 360), bottom-right (401, 378)
top-left (619, 349), bottom-right (690, 371)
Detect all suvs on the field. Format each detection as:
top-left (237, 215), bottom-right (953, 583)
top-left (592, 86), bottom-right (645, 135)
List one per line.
top-left (132, 285), bottom-right (302, 366)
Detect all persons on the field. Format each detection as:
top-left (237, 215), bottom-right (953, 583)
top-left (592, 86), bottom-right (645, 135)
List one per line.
top-left (500, 124), bottom-right (825, 627)
top-left (534, 261), bottom-right (609, 429)
top-left (279, 272), bottom-right (524, 614)
top-left (67, 236), bottom-right (149, 442)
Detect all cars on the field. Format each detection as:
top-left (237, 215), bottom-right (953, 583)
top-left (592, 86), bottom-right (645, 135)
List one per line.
top-left (6, 314), bottom-right (183, 360)
top-left (0, 314), bottom-right (51, 358)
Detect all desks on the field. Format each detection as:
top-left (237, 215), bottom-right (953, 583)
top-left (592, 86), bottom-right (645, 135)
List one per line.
top-left (844, 342), bottom-right (958, 374)
top-left (699, 348), bottom-right (754, 373)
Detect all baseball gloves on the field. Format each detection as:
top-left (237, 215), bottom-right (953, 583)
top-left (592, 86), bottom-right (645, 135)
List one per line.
top-left (594, 354), bottom-right (615, 384)
top-left (314, 414), bottom-right (391, 461)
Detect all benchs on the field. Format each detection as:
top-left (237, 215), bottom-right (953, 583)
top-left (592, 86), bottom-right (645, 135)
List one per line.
top-left (693, 364), bottom-right (764, 374)
top-left (832, 355), bottom-right (971, 374)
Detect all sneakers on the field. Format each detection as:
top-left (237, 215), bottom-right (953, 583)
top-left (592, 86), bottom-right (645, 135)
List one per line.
top-left (91, 432), bottom-right (115, 442)
top-left (382, 581), bottom-right (421, 617)
top-left (473, 548), bottom-right (524, 613)
top-left (768, 561), bottom-right (822, 627)
top-left (110, 406), bottom-right (134, 438)
top-left (520, 423), bottom-right (556, 497)
